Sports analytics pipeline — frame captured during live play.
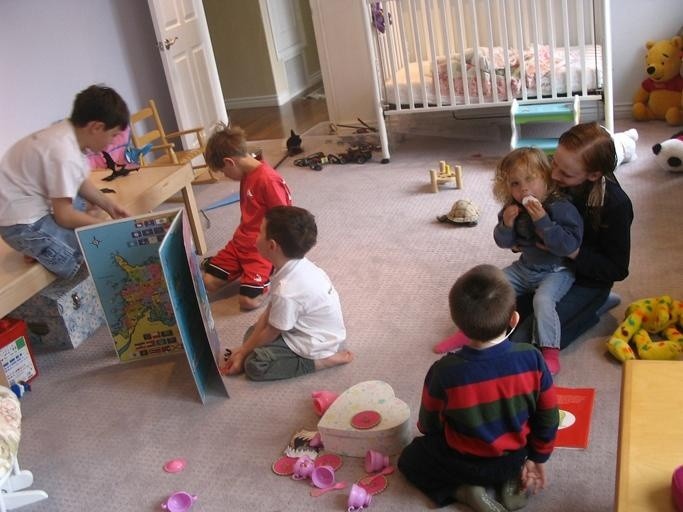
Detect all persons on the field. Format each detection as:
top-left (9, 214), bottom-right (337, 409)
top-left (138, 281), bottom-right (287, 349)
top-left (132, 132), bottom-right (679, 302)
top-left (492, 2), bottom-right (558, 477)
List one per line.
top-left (220, 206), bottom-right (355, 382)
top-left (200, 117), bottom-right (293, 308)
top-left (509, 121), bottom-right (634, 351)
top-left (398, 263), bottom-right (557, 511)
top-left (434, 147), bottom-right (583, 376)
top-left (0, 83), bottom-right (130, 281)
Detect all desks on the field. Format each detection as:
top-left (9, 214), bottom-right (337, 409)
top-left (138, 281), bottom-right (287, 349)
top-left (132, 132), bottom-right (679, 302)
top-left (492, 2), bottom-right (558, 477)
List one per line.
top-left (0, 158), bottom-right (210, 322)
top-left (614, 356), bottom-right (683, 512)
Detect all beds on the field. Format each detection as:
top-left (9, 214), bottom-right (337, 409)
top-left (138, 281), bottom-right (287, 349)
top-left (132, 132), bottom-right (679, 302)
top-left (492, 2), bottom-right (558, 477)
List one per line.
top-left (358, 0), bottom-right (616, 165)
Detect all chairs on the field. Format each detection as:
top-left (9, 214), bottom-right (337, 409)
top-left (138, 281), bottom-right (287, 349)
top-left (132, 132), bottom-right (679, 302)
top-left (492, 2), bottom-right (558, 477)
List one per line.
top-left (125, 96), bottom-right (221, 204)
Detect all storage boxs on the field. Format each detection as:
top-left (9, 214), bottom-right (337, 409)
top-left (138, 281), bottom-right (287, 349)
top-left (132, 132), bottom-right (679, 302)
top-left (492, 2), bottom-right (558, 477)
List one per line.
top-left (3, 267), bottom-right (105, 352)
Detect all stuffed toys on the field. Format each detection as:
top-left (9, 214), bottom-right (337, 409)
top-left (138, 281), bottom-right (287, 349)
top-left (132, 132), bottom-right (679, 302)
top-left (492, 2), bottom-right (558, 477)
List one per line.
top-left (632, 35), bottom-right (683, 127)
top-left (604, 125), bottom-right (638, 165)
top-left (607, 293), bottom-right (683, 361)
top-left (651, 130), bottom-right (682, 172)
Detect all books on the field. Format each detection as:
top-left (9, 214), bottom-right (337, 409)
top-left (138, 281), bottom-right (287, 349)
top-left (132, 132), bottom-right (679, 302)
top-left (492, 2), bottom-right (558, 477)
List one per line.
top-left (551, 385), bottom-right (596, 451)
top-left (72, 208), bottom-right (229, 404)
